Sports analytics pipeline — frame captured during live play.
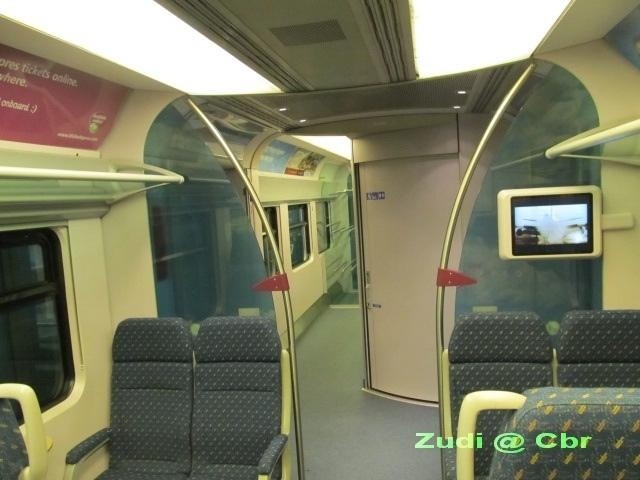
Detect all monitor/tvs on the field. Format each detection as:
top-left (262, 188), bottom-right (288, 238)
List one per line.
top-left (496, 184), bottom-right (605, 262)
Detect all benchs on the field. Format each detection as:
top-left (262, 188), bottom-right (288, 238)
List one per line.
top-left (64, 315), bottom-right (293, 480)
top-left (441, 307), bottom-right (640, 480)
top-left (0, 382), bottom-right (47, 480)
top-left (456, 385), bottom-right (640, 480)
